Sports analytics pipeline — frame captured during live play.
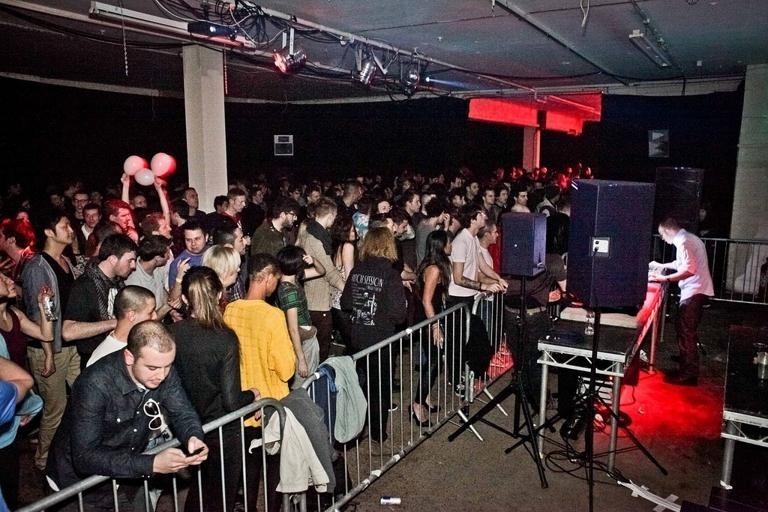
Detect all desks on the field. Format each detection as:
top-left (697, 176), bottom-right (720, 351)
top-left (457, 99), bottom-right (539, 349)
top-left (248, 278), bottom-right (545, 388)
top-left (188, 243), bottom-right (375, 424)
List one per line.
top-left (535, 273), bottom-right (672, 477)
top-left (719, 323), bottom-right (768, 489)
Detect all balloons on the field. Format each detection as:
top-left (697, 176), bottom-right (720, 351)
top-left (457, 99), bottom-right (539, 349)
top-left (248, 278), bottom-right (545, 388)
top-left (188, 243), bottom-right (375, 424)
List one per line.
top-left (151, 153), bottom-right (174, 175)
top-left (123, 157), bottom-right (144, 176)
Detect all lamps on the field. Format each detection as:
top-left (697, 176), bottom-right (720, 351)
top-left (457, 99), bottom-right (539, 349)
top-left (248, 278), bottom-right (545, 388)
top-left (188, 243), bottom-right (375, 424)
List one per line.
top-left (88, 0), bottom-right (258, 52)
top-left (273, 25), bottom-right (424, 94)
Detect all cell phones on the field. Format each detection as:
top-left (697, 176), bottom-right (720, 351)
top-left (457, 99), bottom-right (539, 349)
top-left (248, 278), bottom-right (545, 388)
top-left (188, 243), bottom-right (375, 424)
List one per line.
top-left (186, 446), bottom-right (204, 456)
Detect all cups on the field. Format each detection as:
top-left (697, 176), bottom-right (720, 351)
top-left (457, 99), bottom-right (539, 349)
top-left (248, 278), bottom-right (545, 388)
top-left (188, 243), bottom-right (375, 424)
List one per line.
top-left (756, 351), bottom-right (768, 380)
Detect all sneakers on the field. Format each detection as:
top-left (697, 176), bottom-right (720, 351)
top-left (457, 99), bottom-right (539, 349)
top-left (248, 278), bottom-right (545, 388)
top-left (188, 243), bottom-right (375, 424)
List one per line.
top-left (389, 375), bottom-right (403, 413)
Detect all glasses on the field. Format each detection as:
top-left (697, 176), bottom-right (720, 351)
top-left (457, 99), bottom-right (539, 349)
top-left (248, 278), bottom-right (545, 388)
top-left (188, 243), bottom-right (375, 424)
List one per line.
top-left (143, 396), bottom-right (165, 432)
top-left (283, 210), bottom-right (298, 217)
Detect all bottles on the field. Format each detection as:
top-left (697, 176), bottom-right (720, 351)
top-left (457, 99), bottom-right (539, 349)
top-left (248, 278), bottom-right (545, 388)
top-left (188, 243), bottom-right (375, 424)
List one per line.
top-left (584, 309), bottom-right (595, 337)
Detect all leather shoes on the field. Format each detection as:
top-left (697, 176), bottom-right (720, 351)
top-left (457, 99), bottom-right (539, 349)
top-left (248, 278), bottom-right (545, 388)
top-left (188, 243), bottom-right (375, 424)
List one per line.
top-left (667, 375), bottom-right (699, 387)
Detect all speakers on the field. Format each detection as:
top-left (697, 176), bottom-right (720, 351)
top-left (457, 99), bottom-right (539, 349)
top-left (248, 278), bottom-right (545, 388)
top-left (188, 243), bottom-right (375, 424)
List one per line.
top-left (502, 213), bottom-right (546, 278)
top-left (654, 166), bottom-right (705, 237)
top-left (567, 179), bottom-right (658, 308)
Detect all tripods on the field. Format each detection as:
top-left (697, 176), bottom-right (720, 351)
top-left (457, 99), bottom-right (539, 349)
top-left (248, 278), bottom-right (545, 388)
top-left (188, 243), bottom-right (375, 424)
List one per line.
top-left (505, 307), bottom-right (667, 512)
top-left (448, 277), bottom-right (555, 489)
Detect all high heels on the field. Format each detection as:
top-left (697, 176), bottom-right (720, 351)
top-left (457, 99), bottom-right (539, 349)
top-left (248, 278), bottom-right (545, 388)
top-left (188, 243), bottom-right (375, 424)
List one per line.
top-left (407, 402), bottom-right (434, 428)
top-left (415, 396), bottom-right (442, 413)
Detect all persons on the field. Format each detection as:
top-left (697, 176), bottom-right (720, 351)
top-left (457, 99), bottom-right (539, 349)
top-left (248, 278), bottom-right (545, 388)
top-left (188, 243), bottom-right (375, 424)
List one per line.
top-left (648, 218), bottom-right (714, 384)
